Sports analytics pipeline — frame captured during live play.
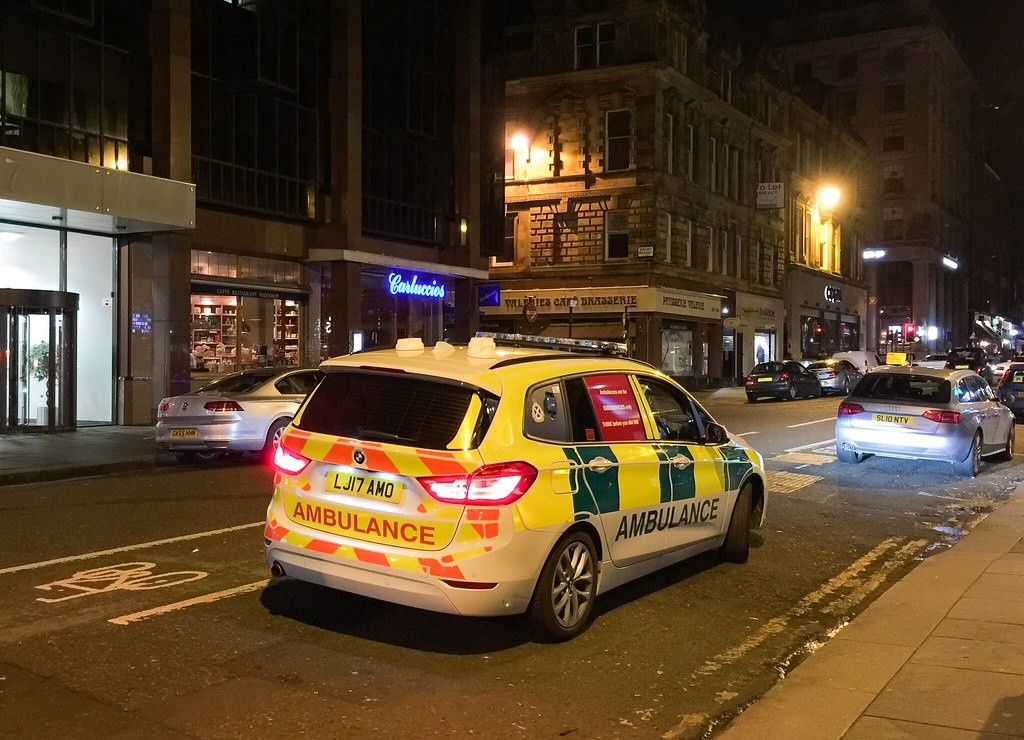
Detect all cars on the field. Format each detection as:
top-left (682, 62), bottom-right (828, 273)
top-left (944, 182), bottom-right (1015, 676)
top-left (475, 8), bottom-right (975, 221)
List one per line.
top-left (744, 360), bottom-right (822, 403)
top-left (155, 366), bottom-right (320, 467)
top-left (806, 358), bottom-right (865, 396)
top-left (990, 354), bottom-right (1024, 376)
top-left (834, 362), bottom-right (1016, 478)
top-left (995, 362), bottom-right (1024, 417)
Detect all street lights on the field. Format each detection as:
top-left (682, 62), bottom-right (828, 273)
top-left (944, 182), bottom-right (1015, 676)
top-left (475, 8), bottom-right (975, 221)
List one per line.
top-left (568, 295), bottom-right (579, 339)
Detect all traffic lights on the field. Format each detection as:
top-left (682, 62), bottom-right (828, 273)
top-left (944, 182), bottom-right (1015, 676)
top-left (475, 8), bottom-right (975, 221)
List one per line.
top-left (905, 323), bottom-right (916, 344)
top-left (887, 330), bottom-right (893, 342)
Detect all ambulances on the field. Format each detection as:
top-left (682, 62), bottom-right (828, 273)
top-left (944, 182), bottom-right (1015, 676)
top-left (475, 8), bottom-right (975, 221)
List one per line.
top-left (264, 331), bottom-right (769, 643)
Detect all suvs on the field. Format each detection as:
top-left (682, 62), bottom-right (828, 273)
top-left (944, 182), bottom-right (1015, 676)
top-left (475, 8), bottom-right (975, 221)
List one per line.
top-left (945, 346), bottom-right (994, 382)
